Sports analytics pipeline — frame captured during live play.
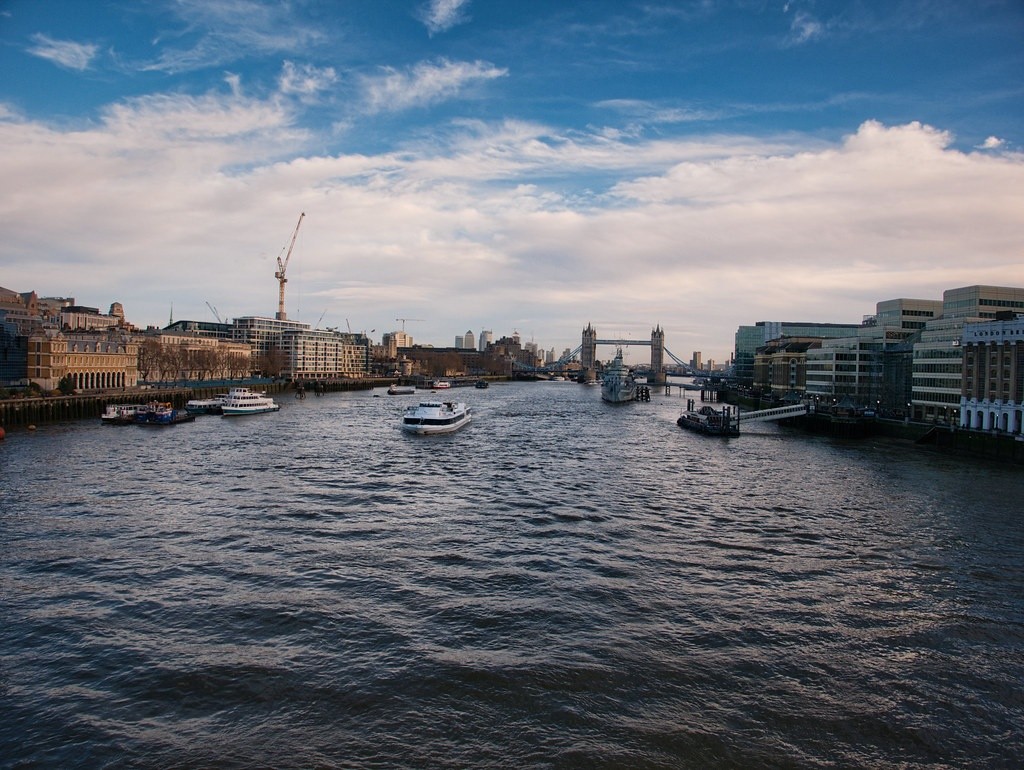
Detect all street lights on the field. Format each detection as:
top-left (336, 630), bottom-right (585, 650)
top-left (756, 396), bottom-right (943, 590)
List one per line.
top-left (996, 413), bottom-right (999, 428)
top-left (832, 397), bottom-right (836, 409)
top-left (737, 383), bottom-right (741, 394)
top-left (793, 390), bottom-right (797, 407)
top-left (743, 385), bottom-right (746, 395)
top-left (943, 404), bottom-right (948, 420)
top-left (749, 386), bottom-right (752, 397)
top-left (783, 388), bottom-right (788, 398)
top-left (907, 402), bottom-right (910, 417)
top-left (952, 408), bottom-right (957, 426)
top-left (816, 396), bottom-right (818, 411)
top-left (798, 393), bottom-right (803, 403)
top-left (875, 397), bottom-right (880, 413)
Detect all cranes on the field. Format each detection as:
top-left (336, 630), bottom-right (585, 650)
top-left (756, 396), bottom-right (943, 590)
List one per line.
top-left (274, 210), bottom-right (305, 323)
top-left (396, 316), bottom-right (427, 329)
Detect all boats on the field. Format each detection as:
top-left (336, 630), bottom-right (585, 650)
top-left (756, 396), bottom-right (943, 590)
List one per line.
top-left (187, 393), bottom-right (228, 413)
top-left (403, 399), bottom-right (472, 433)
top-left (430, 379), bottom-right (450, 390)
top-left (136, 401), bottom-right (194, 426)
top-left (474, 378), bottom-right (489, 389)
top-left (601, 342), bottom-right (638, 405)
top-left (221, 387), bottom-right (280, 416)
top-left (102, 404), bottom-right (147, 423)
top-left (678, 397), bottom-right (743, 440)
top-left (388, 383), bottom-right (415, 395)
